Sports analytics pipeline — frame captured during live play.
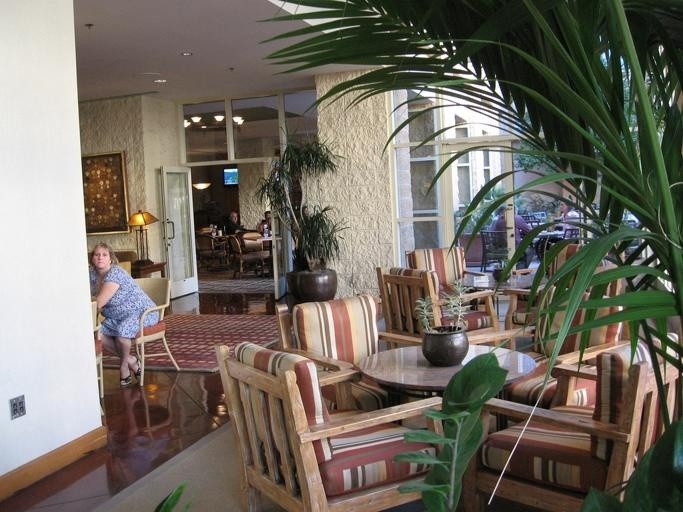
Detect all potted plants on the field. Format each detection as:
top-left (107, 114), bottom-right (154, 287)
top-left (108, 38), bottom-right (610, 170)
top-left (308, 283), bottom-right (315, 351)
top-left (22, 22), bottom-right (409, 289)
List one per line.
top-left (414, 278), bottom-right (473, 368)
top-left (253, 112), bottom-right (350, 301)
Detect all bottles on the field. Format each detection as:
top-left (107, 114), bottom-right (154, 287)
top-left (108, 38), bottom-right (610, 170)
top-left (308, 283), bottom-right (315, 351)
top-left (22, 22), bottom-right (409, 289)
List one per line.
top-left (509, 270), bottom-right (535, 289)
top-left (262, 228), bottom-right (268, 238)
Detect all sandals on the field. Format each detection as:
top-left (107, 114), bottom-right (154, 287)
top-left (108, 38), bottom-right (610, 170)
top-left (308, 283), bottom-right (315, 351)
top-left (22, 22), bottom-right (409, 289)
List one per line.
top-left (120, 372), bottom-right (132, 386)
top-left (134, 355), bottom-right (141, 380)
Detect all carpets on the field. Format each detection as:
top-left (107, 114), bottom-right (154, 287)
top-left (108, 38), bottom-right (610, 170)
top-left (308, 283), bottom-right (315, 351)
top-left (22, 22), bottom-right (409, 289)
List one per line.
top-left (103, 310), bottom-right (279, 373)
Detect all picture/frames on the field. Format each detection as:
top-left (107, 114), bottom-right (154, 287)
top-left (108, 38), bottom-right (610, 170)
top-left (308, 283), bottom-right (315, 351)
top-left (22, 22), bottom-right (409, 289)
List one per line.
top-left (81, 150), bottom-right (132, 236)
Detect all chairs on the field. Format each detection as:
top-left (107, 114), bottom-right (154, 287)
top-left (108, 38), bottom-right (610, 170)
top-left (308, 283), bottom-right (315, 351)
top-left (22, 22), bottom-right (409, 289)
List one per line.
top-left (196, 203), bottom-right (273, 279)
top-left (504, 243), bottom-right (622, 328)
top-left (212, 342), bottom-right (444, 512)
top-left (108, 371), bottom-right (190, 455)
top-left (374, 265), bottom-right (498, 347)
top-left (91, 301), bottom-right (104, 417)
top-left (468, 286), bottom-right (623, 409)
top-left (98, 278), bottom-right (180, 387)
top-left (461, 331), bottom-right (681, 511)
top-left (405, 246), bottom-right (489, 292)
top-left (478, 203), bottom-right (636, 272)
top-left (275, 292), bottom-right (389, 409)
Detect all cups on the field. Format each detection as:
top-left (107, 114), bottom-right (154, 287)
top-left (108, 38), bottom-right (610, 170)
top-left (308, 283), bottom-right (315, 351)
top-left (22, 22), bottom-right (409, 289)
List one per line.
top-left (211, 229), bottom-right (216, 236)
top-left (216, 230), bottom-right (222, 236)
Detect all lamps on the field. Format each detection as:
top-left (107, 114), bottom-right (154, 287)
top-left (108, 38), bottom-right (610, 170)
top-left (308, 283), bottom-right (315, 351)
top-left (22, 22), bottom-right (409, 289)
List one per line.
top-left (184, 111), bottom-right (249, 129)
top-left (125, 210), bottom-right (159, 266)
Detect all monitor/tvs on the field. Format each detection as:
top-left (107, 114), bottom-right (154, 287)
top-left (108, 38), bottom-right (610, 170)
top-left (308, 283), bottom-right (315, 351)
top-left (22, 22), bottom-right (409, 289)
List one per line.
top-left (222, 167), bottom-right (239, 186)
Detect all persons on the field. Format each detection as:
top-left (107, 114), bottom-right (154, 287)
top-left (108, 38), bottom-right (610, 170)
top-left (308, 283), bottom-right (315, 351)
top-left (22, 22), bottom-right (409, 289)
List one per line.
top-left (257, 211), bottom-right (272, 235)
top-left (554, 200), bottom-right (582, 246)
top-left (488, 206), bottom-right (505, 244)
top-left (225, 210), bottom-right (253, 235)
top-left (496, 205), bottom-right (535, 268)
top-left (102, 386), bottom-right (168, 486)
top-left (87, 242), bottom-right (159, 388)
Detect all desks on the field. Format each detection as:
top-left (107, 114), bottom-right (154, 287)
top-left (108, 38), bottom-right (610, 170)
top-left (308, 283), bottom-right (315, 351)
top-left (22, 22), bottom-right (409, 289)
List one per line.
top-left (357, 342), bottom-right (536, 392)
top-left (455, 271), bottom-right (549, 293)
top-left (131, 262), bottom-right (170, 315)
